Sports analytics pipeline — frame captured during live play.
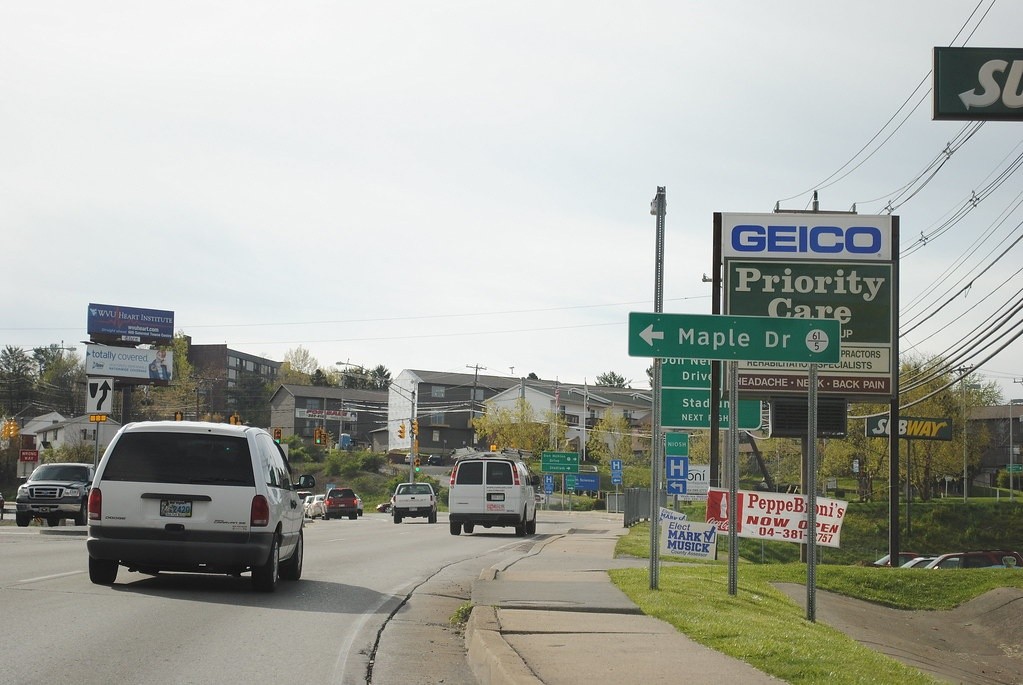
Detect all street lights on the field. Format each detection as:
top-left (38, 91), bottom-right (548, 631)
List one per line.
top-left (335, 361), bottom-right (415, 491)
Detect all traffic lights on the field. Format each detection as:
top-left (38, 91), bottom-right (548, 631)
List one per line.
top-left (412, 421), bottom-right (418, 436)
top-left (1, 420), bottom-right (18, 442)
top-left (416, 458), bottom-right (420, 478)
top-left (313, 428), bottom-right (323, 446)
top-left (273, 427), bottom-right (282, 445)
top-left (398, 424), bottom-right (405, 438)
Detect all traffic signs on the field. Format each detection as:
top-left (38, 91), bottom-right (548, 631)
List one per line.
top-left (628, 312), bottom-right (841, 365)
top-left (541, 450), bottom-right (579, 475)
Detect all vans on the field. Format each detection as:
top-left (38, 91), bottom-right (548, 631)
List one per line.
top-left (448, 455), bottom-right (540, 536)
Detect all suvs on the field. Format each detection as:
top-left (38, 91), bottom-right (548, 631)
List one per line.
top-left (15, 462), bottom-right (97, 529)
top-left (320, 489), bottom-right (358, 520)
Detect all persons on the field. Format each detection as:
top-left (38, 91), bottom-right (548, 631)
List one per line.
top-left (149, 350), bottom-right (170, 380)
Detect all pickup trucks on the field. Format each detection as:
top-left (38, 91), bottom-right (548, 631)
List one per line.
top-left (392, 483), bottom-right (439, 524)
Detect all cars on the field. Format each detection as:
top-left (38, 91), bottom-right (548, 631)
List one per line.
top-left (85, 420), bottom-right (316, 590)
top-left (874, 552), bottom-right (1022, 568)
top-left (377, 495), bottom-right (395, 514)
top-left (405, 453), bottom-right (420, 464)
top-left (354, 495), bottom-right (363, 516)
top-left (428, 454), bottom-right (444, 465)
top-left (296, 491), bottom-right (326, 520)
